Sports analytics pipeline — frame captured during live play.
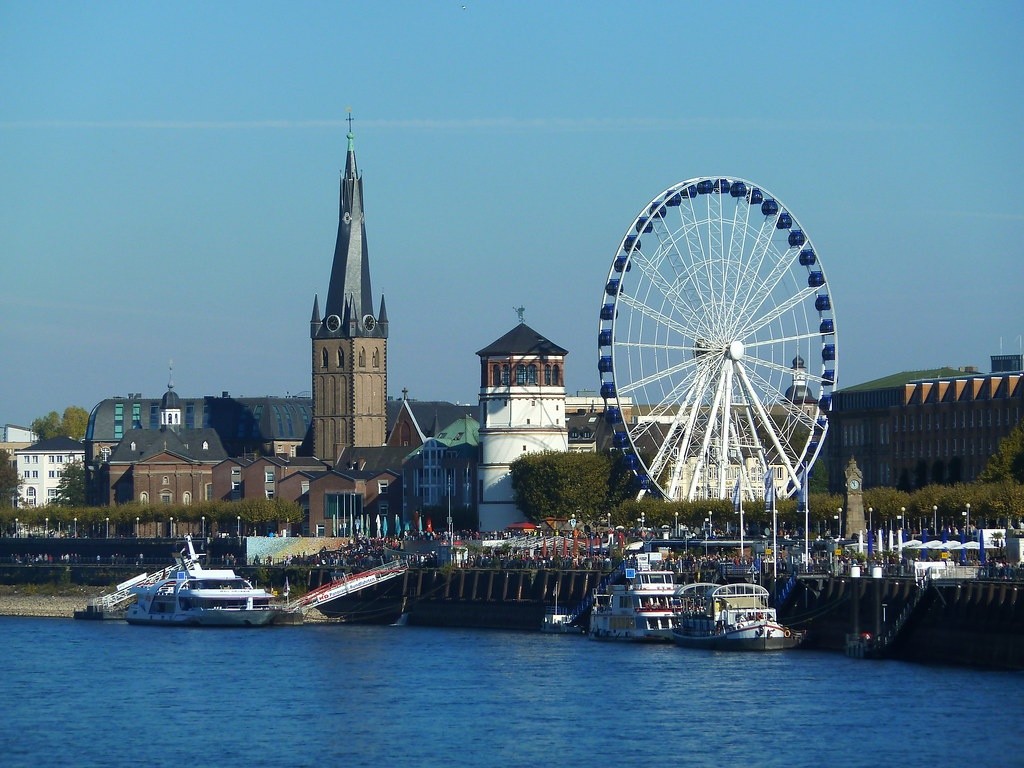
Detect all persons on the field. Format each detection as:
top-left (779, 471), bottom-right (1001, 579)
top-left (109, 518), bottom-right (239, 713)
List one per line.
top-left (0, 521), bottom-right (1024, 579)
top-left (598, 596), bottom-right (774, 636)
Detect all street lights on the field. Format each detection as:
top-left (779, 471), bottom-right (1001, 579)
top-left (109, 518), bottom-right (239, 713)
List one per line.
top-left (202, 516), bottom-right (205, 538)
top-left (933, 505), bottom-right (937, 535)
top-left (136, 516), bottom-right (140, 537)
top-left (45, 518), bottom-right (49, 537)
top-left (868, 507), bottom-right (873, 530)
top-left (237, 515), bottom-right (241, 536)
top-left (641, 512), bottom-right (645, 531)
top-left (675, 512), bottom-right (678, 537)
top-left (15, 517), bottom-right (18, 538)
top-left (708, 510), bottom-right (712, 537)
top-left (106, 517), bottom-right (109, 538)
top-left (901, 507), bottom-right (906, 528)
top-left (837, 507), bottom-right (843, 538)
top-left (607, 513), bottom-right (611, 529)
top-left (170, 516), bottom-right (173, 539)
top-left (965, 502), bottom-right (971, 535)
top-left (74, 517), bottom-right (77, 539)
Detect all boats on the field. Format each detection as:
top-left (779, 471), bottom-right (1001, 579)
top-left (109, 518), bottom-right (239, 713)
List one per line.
top-left (669, 582), bottom-right (807, 652)
top-left (126, 534), bottom-right (284, 628)
top-left (587, 563), bottom-right (712, 640)
